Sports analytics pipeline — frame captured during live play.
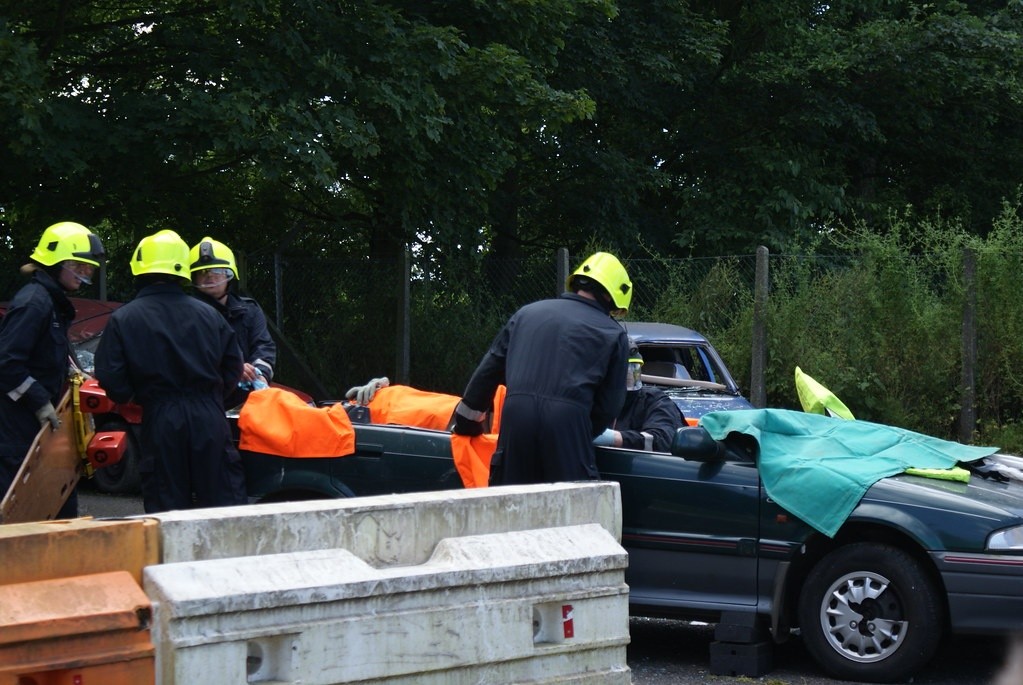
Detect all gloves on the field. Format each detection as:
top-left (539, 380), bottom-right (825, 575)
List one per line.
top-left (345, 377), bottom-right (390, 406)
top-left (69, 365), bottom-right (92, 380)
top-left (35, 400), bottom-right (60, 429)
top-left (593, 429), bottom-right (615, 447)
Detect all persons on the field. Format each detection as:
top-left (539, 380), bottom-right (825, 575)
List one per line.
top-left (189, 236), bottom-right (276, 411)
top-left (450, 251), bottom-right (633, 488)
top-left (591, 336), bottom-right (690, 453)
top-left (0, 221), bottom-right (106, 521)
top-left (93, 228), bottom-right (244, 513)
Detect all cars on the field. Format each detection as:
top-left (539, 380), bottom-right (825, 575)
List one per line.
top-left (63, 299), bottom-right (318, 498)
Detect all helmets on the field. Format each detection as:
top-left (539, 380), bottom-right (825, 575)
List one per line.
top-left (29, 222), bottom-right (104, 268)
top-left (628, 353), bottom-right (644, 368)
top-left (129, 230), bottom-right (191, 284)
top-left (189, 236), bottom-right (239, 281)
top-left (565, 252), bottom-right (633, 317)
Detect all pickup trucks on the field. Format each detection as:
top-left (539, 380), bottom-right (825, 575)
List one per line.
top-left (232, 321), bottom-right (1023, 685)
